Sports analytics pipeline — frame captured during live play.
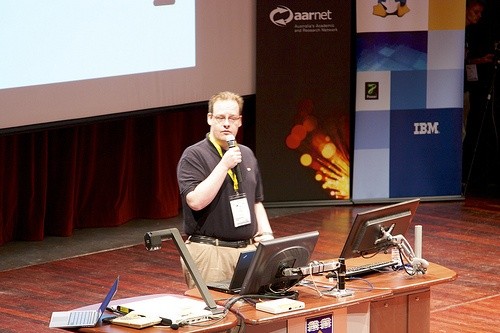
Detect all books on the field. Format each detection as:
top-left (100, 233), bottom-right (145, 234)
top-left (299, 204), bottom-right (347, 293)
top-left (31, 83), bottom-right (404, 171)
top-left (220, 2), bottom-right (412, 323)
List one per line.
top-left (111, 310), bottom-right (161, 329)
top-left (256, 298), bottom-right (305, 314)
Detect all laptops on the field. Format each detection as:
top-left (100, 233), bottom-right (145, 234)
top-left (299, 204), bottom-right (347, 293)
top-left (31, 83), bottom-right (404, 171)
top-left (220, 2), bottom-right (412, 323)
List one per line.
top-left (49, 276), bottom-right (120, 328)
top-left (206, 250), bottom-right (257, 295)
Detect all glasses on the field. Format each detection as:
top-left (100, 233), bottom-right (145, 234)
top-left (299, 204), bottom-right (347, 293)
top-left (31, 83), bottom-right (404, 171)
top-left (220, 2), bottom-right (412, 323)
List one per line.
top-left (210, 114), bottom-right (243, 122)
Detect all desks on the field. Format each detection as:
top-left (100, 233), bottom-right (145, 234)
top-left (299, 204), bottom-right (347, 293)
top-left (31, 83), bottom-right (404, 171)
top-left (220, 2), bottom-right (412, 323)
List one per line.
top-left (63, 253), bottom-right (458, 333)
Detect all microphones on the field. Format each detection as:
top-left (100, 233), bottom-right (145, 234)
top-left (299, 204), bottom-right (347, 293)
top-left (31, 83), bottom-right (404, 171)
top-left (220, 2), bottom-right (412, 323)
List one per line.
top-left (225, 134), bottom-right (242, 183)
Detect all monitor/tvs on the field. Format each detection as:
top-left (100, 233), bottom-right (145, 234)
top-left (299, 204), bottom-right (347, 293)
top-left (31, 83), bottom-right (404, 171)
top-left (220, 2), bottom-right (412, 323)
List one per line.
top-left (339, 199), bottom-right (420, 260)
top-left (239, 231), bottom-right (320, 296)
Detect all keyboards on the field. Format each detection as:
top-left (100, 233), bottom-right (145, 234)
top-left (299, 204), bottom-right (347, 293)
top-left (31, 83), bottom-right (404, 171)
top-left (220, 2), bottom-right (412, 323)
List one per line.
top-left (326, 261), bottom-right (398, 279)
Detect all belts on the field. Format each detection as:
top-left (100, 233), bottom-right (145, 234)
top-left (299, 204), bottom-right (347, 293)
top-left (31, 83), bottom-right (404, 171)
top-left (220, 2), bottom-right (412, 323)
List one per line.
top-left (189, 235), bottom-right (254, 249)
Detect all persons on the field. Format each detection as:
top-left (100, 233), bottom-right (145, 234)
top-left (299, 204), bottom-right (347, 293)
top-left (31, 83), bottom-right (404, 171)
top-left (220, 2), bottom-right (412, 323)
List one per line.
top-left (177, 91), bottom-right (274, 290)
